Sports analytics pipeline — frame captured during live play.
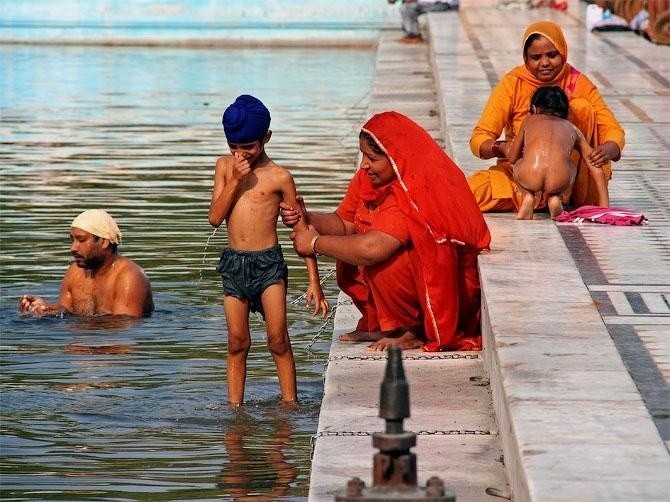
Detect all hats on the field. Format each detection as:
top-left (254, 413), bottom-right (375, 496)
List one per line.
top-left (72, 209), bottom-right (121, 245)
top-left (222, 94), bottom-right (270, 143)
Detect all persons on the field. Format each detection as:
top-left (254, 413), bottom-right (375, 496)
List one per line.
top-left (21, 209), bottom-right (154, 318)
top-left (280, 112), bottom-right (491, 353)
top-left (466, 21), bottom-right (625, 212)
top-left (505, 86), bottom-right (609, 219)
top-left (216, 405), bottom-right (291, 502)
top-left (208, 94), bottom-right (330, 405)
top-left (388, 0), bottom-right (458, 44)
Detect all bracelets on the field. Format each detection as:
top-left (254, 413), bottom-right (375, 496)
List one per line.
top-left (492, 141), bottom-right (508, 158)
top-left (311, 235), bottom-right (322, 256)
